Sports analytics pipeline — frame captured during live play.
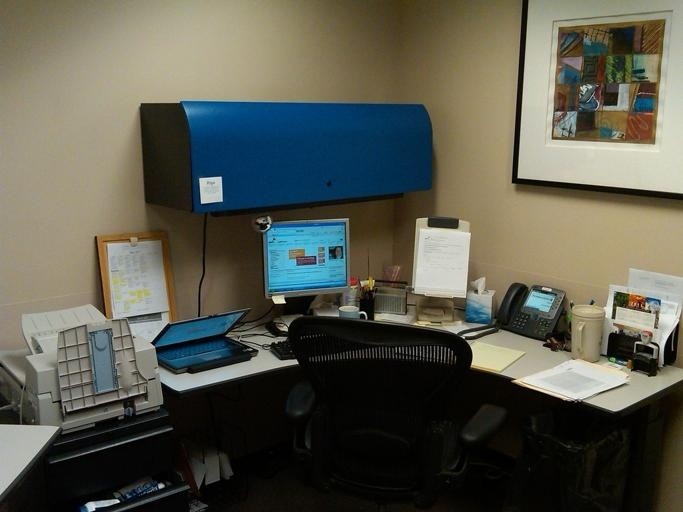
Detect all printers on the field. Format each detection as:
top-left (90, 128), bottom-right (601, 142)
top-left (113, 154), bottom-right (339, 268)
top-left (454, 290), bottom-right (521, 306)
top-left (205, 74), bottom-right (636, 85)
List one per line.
top-left (0, 304), bottom-right (166, 436)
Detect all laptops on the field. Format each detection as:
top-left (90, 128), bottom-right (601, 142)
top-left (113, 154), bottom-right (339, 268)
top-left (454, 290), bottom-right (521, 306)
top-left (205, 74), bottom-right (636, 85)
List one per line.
top-left (152, 308), bottom-right (259, 375)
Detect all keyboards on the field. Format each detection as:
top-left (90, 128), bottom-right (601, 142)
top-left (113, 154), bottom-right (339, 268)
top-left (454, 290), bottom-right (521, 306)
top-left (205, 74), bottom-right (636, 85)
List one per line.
top-left (270, 336), bottom-right (369, 360)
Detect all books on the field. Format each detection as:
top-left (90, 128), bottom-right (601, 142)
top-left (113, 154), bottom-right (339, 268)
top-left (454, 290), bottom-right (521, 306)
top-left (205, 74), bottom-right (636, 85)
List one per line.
top-left (466, 341), bottom-right (526, 373)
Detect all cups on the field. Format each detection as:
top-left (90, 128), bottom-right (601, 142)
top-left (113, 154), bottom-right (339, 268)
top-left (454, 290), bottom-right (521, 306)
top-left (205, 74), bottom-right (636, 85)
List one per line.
top-left (571, 304), bottom-right (605, 363)
top-left (337, 305), bottom-right (367, 319)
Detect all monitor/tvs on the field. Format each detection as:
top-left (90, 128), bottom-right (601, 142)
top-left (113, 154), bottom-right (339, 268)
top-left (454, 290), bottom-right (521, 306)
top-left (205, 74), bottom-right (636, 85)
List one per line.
top-left (261, 217), bottom-right (350, 336)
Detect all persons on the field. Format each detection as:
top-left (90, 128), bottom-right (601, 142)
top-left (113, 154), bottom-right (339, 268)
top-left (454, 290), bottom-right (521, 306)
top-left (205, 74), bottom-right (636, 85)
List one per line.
top-left (334, 246), bottom-right (343, 259)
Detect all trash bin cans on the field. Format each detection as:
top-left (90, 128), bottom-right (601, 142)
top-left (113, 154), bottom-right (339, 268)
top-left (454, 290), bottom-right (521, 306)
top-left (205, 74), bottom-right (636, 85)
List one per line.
top-left (522, 409), bottom-right (629, 512)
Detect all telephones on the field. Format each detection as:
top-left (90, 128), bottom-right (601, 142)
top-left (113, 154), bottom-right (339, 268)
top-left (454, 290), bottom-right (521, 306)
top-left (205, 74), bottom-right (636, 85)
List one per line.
top-left (495, 282), bottom-right (566, 342)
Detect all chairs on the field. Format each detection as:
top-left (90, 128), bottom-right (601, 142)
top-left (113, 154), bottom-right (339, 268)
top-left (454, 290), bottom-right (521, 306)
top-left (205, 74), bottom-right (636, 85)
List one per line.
top-left (285, 315), bottom-right (508, 512)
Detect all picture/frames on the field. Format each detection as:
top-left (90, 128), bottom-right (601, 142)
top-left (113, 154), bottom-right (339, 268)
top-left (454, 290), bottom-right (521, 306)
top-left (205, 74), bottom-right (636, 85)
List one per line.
top-left (512, 0), bottom-right (683, 200)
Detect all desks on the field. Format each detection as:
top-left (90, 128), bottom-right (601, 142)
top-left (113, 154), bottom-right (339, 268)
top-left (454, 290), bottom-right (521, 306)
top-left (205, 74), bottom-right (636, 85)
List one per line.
top-left (0, 424), bottom-right (61, 512)
top-left (159, 305), bottom-right (682, 512)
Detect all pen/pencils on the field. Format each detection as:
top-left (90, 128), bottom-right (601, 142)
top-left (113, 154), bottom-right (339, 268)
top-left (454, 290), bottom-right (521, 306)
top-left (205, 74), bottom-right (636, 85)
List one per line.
top-left (590, 299), bottom-right (595, 305)
top-left (570, 300), bottom-right (573, 309)
top-left (359, 276), bottom-right (378, 300)
